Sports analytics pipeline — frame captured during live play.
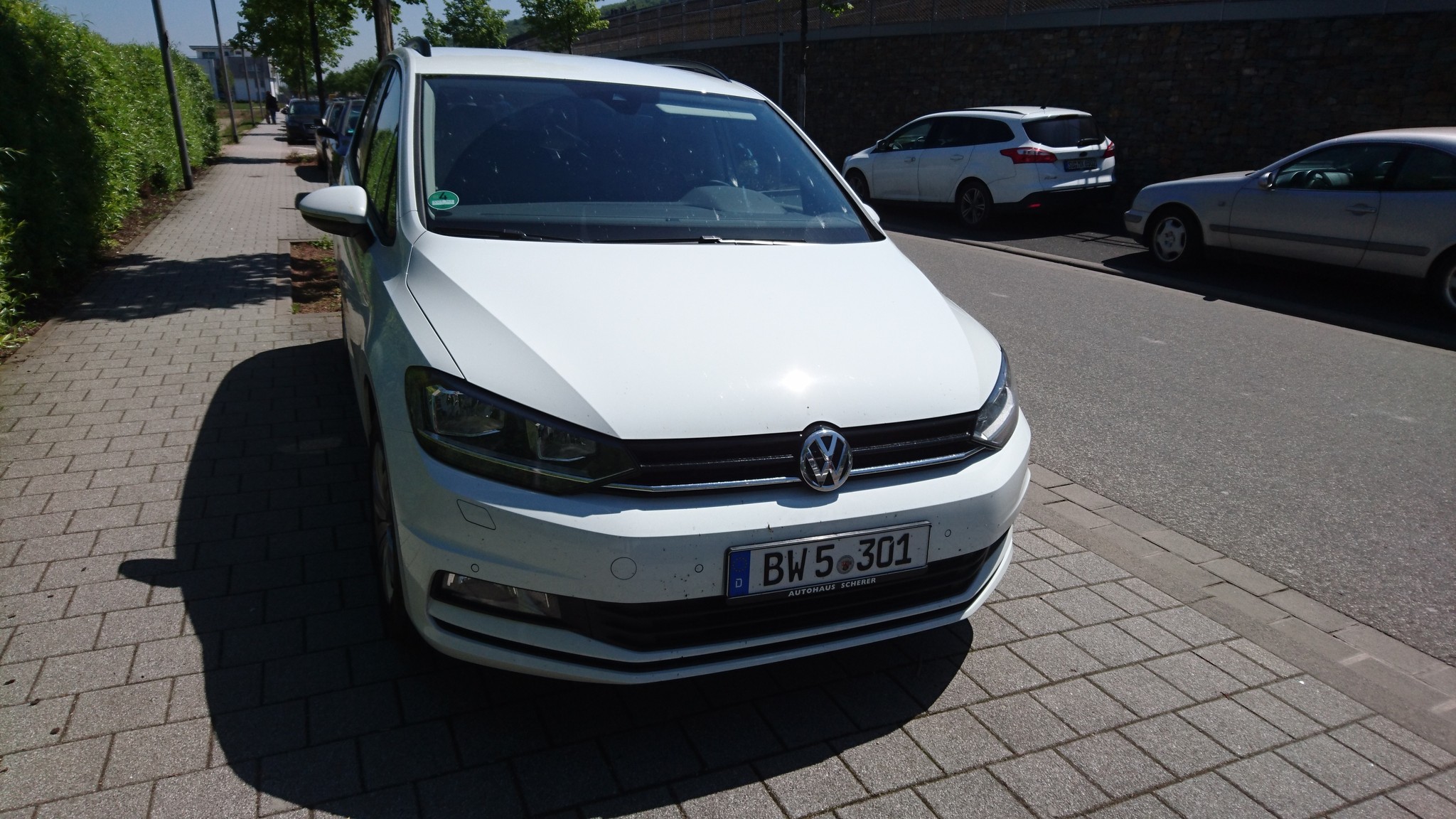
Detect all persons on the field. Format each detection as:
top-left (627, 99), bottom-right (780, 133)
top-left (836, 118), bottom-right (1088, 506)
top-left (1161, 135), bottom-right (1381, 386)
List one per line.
top-left (265, 91), bottom-right (277, 124)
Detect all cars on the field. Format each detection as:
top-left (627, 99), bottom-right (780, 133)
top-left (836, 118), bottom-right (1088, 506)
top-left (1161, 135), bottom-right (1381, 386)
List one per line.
top-left (284, 98), bottom-right (317, 143)
top-left (1123, 125), bottom-right (1455, 310)
top-left (298, 34), bottom-right (1034, 686)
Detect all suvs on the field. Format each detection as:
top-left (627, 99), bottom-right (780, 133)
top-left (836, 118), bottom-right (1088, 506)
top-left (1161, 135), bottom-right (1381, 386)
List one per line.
top-left (315, 96), bottom-right (367, 187)
top-left (846, 101), bottom-right (1115, 231)
